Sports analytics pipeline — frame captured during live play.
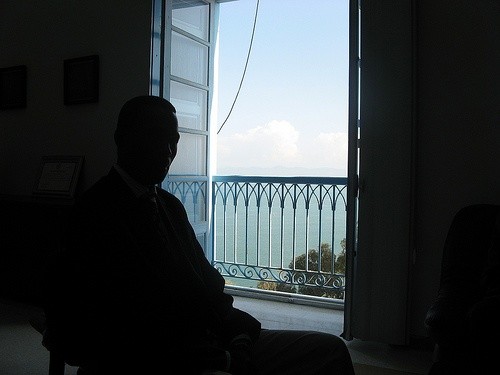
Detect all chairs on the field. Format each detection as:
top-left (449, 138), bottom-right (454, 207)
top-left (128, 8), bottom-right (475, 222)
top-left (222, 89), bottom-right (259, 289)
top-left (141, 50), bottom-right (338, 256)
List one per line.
top-left (35, 207), bottom-right (82, 374)
top-left (429, 204), bottom-right (500, 375)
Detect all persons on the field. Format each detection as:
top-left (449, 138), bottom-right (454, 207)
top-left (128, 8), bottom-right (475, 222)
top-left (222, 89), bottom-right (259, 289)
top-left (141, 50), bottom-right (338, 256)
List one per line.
top-left (30, 93), bottom-right (357, 375)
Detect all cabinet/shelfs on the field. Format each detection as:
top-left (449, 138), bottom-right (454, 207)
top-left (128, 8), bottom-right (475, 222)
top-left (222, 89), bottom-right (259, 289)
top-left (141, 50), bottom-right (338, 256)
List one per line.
top-left (0, 192), bottom-right (85, 300)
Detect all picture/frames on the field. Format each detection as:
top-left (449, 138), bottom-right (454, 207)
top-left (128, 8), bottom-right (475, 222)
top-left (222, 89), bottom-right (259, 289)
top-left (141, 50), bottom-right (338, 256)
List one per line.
top-left (30, 153), bottom-right (83, 200)
top-left (1, 63), bottom-right (28, 110)
top-left (62, 54), bottom-right (101, 105)
